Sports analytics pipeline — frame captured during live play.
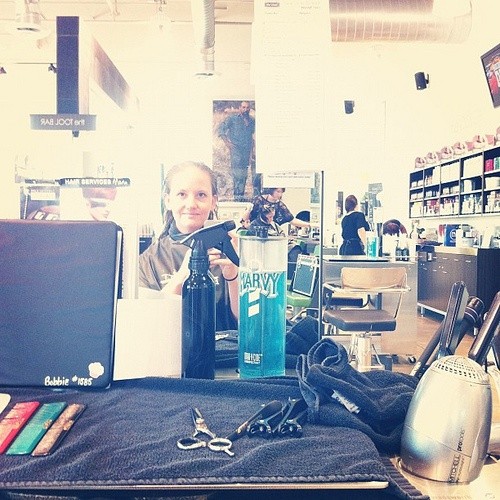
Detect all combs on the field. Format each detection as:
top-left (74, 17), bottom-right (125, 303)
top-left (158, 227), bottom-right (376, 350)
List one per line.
top-left (0, 401), bottom-right (88, 456)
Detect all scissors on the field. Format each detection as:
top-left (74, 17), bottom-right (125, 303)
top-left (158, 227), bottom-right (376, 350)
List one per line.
top-left (176, 406), bottom-right (235, 458)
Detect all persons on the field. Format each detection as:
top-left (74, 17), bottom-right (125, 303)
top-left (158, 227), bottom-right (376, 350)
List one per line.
top-left (217, 102), bottom-right (256, 201)
top-left (139, 160), bottom-right (239, 332)
top-left (339, 195), bottom-right (371, 255)
top-left (240, 187), bottom-right (310, 237)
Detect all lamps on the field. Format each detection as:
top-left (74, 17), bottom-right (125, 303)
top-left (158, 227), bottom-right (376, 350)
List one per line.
top-left (415, 72), bottom-right (429, 90)
top-left (344, 100), bottom-right (354, 115)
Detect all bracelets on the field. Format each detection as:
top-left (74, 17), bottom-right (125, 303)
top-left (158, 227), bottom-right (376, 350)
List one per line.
top-left (225, 273), bottom-right (238, 281)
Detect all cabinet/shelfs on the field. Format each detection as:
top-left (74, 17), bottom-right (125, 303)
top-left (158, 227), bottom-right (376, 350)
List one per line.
top-left (416, 244), bottom-right (500, 336)
top-left (409, 144), bottom-right (500, 220)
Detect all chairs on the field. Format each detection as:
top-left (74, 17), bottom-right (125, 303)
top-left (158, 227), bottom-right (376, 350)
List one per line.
top-left (324, 267), bottom-right (411, 370)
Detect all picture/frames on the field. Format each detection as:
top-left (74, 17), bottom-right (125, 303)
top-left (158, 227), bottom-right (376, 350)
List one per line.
top-left (480, 43), bottom-right (500, 109)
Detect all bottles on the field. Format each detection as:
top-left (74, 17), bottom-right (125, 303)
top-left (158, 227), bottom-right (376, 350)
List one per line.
top-left (456, 228), bottom-right (463, 248)
top-left (237, 225), bottom-right (289, 380)
top-left (181, 239), bottom-right (216, 380)
top-left (367, 228), bottom-right (377, 259)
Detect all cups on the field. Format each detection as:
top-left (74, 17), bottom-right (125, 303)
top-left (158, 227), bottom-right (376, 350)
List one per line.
top-left (494, 157), bottom-right (500, 170)
top-left (485, 159), bottom-right (494, 172)
